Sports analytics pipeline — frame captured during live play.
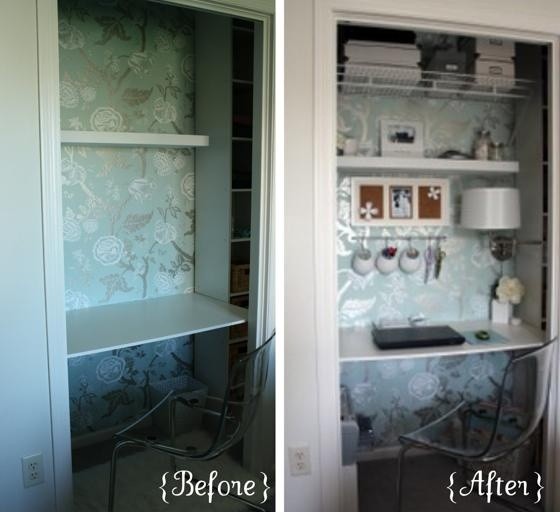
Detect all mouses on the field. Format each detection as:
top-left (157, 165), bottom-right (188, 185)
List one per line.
top-left (476, 330), bottom-right (491, 340)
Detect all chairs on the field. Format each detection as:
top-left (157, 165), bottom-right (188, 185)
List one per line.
top-left (395, 338), bottom-right (554, 510)
top-left (105, 334), bottom-right (277, 511)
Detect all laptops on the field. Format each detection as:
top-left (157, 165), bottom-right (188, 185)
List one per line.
top-left (372, 327), bottom-right (463, 349)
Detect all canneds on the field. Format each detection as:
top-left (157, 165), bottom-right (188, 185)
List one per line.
top-left (475, 128), bottom-right (492, 160)
top-left (489, 143), bottom-right (503, 161)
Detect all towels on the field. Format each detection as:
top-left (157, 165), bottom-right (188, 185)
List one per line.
top-left (338, 24), bottom-right (421, 97)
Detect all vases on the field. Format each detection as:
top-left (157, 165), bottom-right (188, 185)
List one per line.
top-left (491, 299), bottom-right (513, 323)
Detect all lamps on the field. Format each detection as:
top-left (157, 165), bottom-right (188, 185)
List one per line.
top-left (459, 185), bottom-right (522, 261)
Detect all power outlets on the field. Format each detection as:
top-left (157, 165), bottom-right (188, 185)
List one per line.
top-left (21, 453), bottom-right (45, 488)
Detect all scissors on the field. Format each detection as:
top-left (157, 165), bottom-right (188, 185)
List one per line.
top-left (424, 248), bottom-right (445, 283)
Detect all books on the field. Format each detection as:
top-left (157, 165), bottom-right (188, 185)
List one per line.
top-left (341, 40), bottom-right (422, 98)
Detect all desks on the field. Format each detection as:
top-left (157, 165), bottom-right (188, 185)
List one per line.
top-left (339, 322), bottom-right (543, 361)
top-left (68, 290), bottom-right (250, 356)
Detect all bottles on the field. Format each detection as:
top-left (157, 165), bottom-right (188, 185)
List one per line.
top-left (472, 129), bottom-right (505, 161)
top-left (353, 248), bottom-right (421, 275)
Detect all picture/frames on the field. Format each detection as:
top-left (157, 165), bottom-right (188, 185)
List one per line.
top-left (351, 178), bottom-right (451, 226)
top-left (380, 119), bottom-right (425, 154)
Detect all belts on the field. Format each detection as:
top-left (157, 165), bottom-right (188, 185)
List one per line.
top-left (449, 415), bottom-right (533, 483)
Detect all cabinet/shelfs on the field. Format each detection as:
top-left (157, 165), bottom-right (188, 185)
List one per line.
top-left (335, 61), bottom-right (539, 176)
top-left (194, 10), bottom-right (254, 438)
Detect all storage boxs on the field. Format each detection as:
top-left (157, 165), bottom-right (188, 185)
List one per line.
top-left (427, 36), bottom-right (524, 97)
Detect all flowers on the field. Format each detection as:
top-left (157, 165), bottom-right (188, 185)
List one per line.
top-left (496, 274), bottom-right (526, 306)
